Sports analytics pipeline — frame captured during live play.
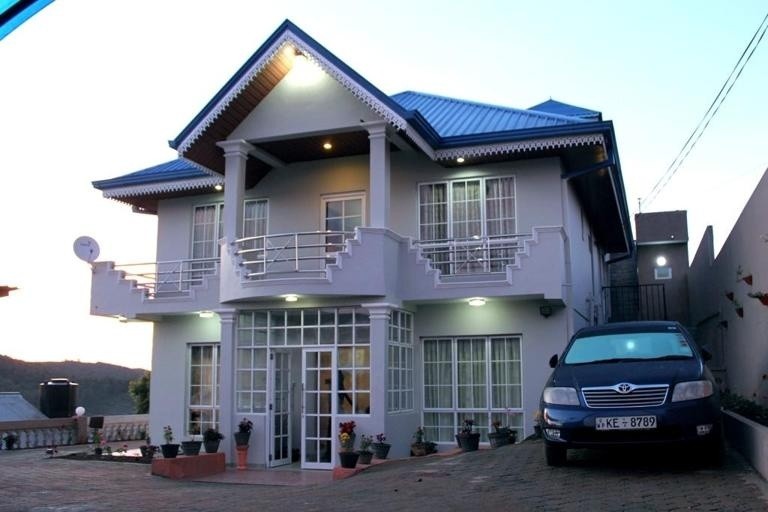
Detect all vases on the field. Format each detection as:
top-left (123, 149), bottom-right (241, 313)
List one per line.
top-left (180, 441), bottom-right (201, 455)
top-left (95, 448), bottom-right (101, 454)
top-left (455, 435), bottom-right (463, 448)
top-left (337, 451), bottom-right (360, 469)
top-left (159, 444), bottom-right (179, 458)
top-left (372, 444), bottom-right (392, 459)
top-left (338, 433), bottom-right (356, 449)
top-left (533, 425), bottom-right (543, 438)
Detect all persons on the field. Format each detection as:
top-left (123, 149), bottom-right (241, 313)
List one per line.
top-left (324, 370), bottom-right (359, 463)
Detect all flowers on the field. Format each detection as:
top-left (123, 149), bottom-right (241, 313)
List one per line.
top-left (338, 420), bottom-right (356, 434)
top-left (164, 426), bottom-right (174, 444)
top-left (118, 444), bottom-right (128, 453)
top-left (376, 433), bottom-right (386, 444)
top-left (339, 433), bottom-right (355, 453)
top-left (94, 430), bottom-right (105, 449)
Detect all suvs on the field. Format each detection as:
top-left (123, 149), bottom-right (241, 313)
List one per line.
top-left (536, 320), bottom-right (727, 470)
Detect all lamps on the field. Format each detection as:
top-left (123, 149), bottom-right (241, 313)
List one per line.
top-left (199, 311), bottom-right (214, 318)
top-left (469, 298), bottom-right (486, 307)
top-left (292, 48), bottom-right (306, 66)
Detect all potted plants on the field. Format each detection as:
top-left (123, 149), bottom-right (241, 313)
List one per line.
top-left (457, 420), bottom-right (479, 450)
top-left (502, 426), bottom-right (517, 445)
top-left (486, 421), bottom-right (504, 449)
top-left (203, 429), bottom-right (224, 453)
top-left (234, 418), bottom-right (253, 446)
top-left (140, 434), bottom-right (156, 459)
top-left (410, 427), bottom-right (429, 457)
top-left (355, 434), bottom-right (374, 464)
